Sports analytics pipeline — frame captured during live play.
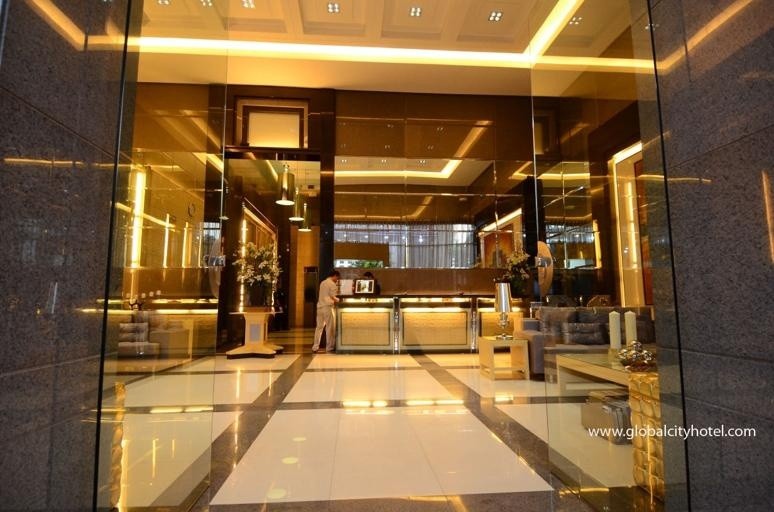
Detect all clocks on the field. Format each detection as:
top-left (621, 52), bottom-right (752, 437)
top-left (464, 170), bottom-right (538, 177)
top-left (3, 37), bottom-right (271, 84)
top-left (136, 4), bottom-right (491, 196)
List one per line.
top-left (188, 204), bottom-right (197, 217)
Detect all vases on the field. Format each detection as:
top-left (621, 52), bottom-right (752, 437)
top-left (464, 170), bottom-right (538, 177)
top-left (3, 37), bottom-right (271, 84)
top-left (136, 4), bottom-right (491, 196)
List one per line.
top-left (511, 299), bottom-right (528, 316)
top-left (249, 286), bottom-right (268, 306)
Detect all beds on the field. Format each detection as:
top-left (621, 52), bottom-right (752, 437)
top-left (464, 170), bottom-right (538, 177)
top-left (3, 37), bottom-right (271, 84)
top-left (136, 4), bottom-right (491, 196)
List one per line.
top-left (557, 355), bottom-right (656, 398)
top-left (479, 337), bottom-right (530, 380)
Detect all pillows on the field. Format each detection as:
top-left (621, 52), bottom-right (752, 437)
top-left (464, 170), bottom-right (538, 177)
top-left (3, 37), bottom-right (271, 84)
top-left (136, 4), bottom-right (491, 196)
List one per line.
top-left (607, 322), bottom-right (649, 344)
top-left (562, 322), bottom-right (604, 345)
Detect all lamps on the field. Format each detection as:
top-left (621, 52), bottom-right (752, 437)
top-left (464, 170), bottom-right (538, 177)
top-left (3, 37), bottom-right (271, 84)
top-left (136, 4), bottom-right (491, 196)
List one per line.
top-left (494, 283), bottom-right (512, 339)
top-left (275, 162), bottom-right (311, 231)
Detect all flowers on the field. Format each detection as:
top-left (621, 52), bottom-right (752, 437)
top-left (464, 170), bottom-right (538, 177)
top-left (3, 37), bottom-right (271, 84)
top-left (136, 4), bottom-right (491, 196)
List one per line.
top-left (232, 239), bottom-right (284, 286)
top-left (493, 244), bottom-right (530, 298)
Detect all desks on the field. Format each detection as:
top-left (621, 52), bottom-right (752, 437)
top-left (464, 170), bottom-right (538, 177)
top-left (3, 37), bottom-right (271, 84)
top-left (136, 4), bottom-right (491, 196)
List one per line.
top-left (227, 312), bottom-right (281, 358)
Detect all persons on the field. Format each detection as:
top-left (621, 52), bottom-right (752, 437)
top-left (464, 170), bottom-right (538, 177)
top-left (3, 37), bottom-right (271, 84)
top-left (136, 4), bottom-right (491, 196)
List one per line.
top-left (364, 272), bottom-right (380, 295)
top-left (312, 270), bottom-right (341, 353)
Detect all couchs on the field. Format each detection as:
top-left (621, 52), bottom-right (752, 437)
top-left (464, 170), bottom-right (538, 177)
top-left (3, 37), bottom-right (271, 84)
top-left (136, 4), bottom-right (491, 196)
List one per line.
top-left (513, 307), bottom-right (655, 374)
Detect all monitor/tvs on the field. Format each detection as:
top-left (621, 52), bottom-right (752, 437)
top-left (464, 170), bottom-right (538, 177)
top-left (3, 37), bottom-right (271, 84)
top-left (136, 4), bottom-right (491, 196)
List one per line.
top-left (354, 279), bottom-right (375, 294)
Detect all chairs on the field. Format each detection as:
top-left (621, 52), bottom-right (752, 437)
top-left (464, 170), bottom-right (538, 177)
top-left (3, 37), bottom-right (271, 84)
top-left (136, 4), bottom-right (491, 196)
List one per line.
top-left (118, 323), bottom-right (159, 356)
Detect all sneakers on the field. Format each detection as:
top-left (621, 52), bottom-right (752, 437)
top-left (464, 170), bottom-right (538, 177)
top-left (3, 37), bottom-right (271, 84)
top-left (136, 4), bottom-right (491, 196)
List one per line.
top-left (312, 348), bottom-right (336, 353)
